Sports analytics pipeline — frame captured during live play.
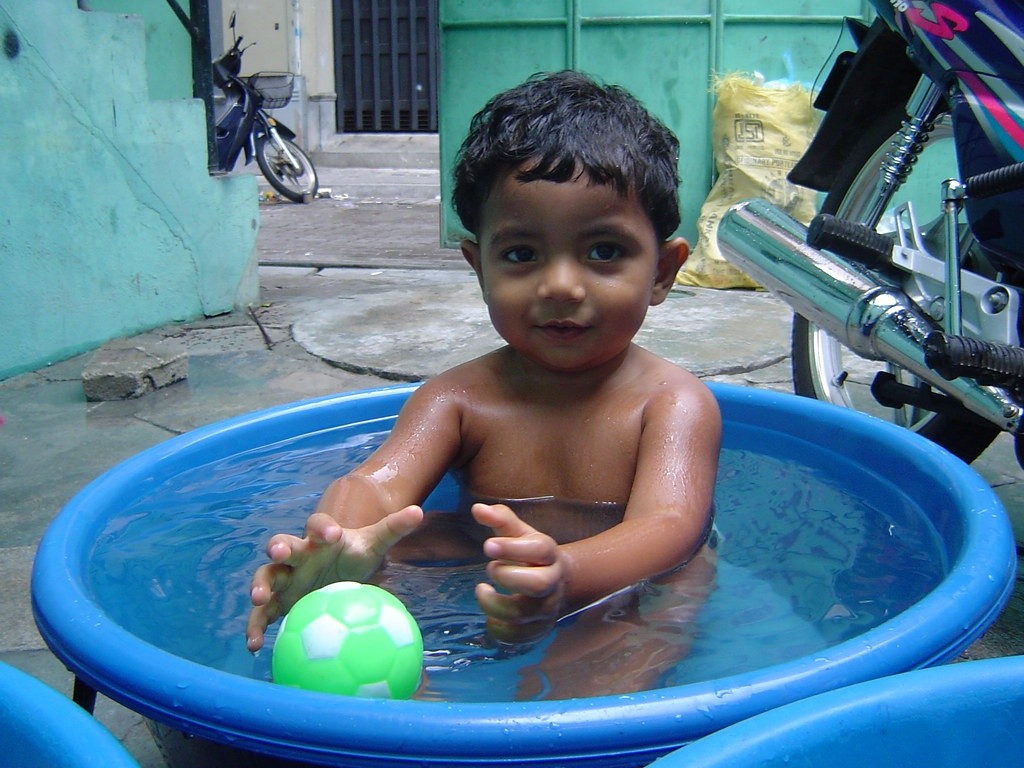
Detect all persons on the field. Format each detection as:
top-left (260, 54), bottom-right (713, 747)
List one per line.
top-left (247, 69), bottom-right (723, 652)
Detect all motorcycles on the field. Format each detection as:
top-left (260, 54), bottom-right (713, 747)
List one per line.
top-left (715, 0), bottom-right (1024, 479)
top-left (211, 10), bottom-right (320, 203)
